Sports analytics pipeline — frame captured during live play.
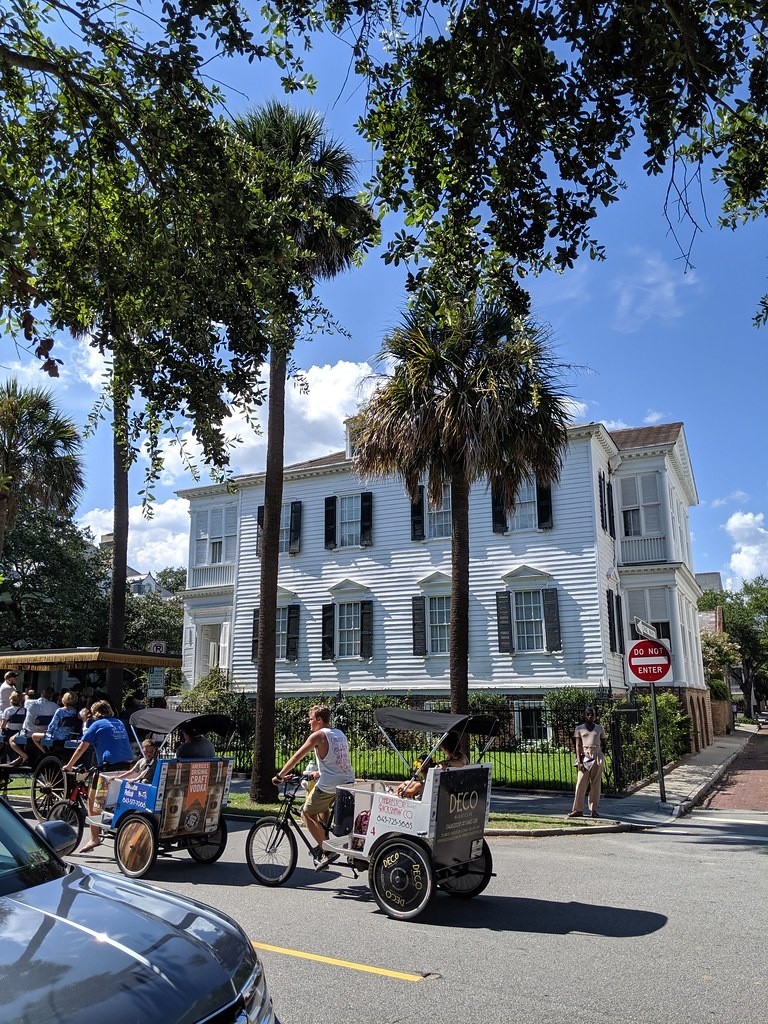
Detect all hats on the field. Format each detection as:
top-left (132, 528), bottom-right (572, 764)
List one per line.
top-left (4, 670), bottom-right (20, 678)
top-left (9, 690), bottom-right (23, 701)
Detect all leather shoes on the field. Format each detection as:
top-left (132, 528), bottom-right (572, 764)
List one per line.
top-left (590, 809), bottom-right (600, 818)
top-left (568, 809), bottom-right (583, 817)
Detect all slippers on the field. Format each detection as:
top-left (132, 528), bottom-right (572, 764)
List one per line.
top-left (79, 841), bottom-right (104, 853)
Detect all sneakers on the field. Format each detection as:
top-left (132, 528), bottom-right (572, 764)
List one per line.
top-left (315, 851), bottom-right (341, 872)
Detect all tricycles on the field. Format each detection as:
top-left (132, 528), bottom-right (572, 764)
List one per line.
top-left (47, 706), bottom-right (241, 880)
top-left (245, 707), bottom-right (498, 918)
top-left (0, 715), bottom-right (98, 822)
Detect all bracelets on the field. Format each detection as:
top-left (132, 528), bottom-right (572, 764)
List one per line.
top-left (275, 772), bottom-right (284, 780)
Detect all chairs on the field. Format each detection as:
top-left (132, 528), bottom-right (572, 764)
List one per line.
top-left (0, 714), bottom-right (92, 767)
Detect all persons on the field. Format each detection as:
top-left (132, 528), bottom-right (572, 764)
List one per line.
top-left (63, 700), bottom-right (136, 855)
top-left (732, 702), bottom-right (738, 721)
top-left (31, 690), bottom-right (84, 756)
top-left (0, 671), bottom-right (20, 752)
top-left (23, 680), bottom-right (171, 725)
top-left (0, 691), bottom-right (26, 760)
top-left (568, 707), bottom-right (611, 817)
top-left (111, 739), bottom-right (159, 786)
top-left (435, 733), bottom-right (470, 767)
top-left (272, 706), bottom-right (357, 870)
top-left (174, 725), bottom-right (216, 758)
top-left (8, 686), bottom-right (61, 767)
top-left (398, 754), bottom-right (434, 800)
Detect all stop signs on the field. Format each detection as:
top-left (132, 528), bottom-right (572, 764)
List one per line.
top-left (627, 640), bottom-right (672, 684)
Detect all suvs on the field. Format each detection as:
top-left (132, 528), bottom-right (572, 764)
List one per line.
top-left (1, 793), bottom-right (283, 1023)
